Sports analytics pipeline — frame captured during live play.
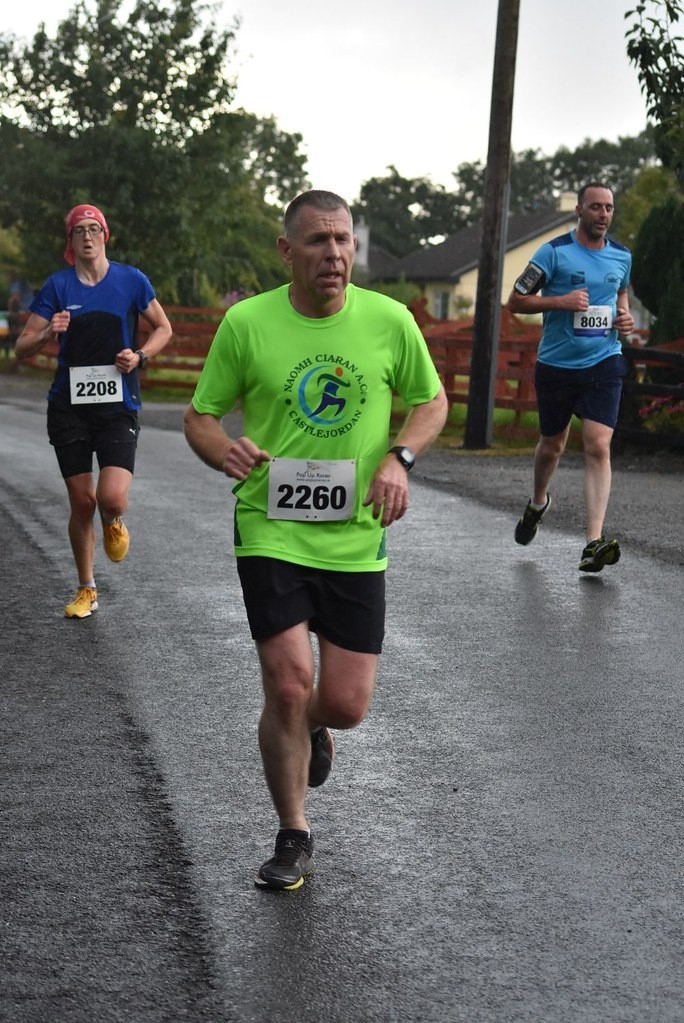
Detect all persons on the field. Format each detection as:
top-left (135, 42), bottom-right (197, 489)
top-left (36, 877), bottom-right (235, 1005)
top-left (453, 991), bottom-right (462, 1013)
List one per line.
top-left (185, 192), bottom-right (450, 892)
top-left (14, 204), bottom-right (172, 618)
top-left (507, 182), bottom-right (636, 573)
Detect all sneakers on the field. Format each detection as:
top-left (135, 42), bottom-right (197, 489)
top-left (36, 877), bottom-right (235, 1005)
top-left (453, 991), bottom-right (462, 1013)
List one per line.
top-left (578, 535), bottom-right (621, 572)
top-left (308, 726), bottom-right (335, 789)
top-left (514, 493), bottom-right (551, 547)
top-left (254, 829), bottom-right (314, 889)
top-left (64, 586), bottom-right (98, 619)
top-left (103, 513), bottom-right (130, 562)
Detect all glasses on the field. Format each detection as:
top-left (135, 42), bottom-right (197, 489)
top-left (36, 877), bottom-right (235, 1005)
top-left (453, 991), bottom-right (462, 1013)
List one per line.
top-left (71, 228), bottom-right (104, 237)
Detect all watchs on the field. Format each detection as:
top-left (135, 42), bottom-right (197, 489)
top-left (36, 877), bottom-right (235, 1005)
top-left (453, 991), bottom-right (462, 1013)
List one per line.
top-left (135, 350), bottom-right (147, 369)
top-left (386, 446), bottom-right (416, 471)
